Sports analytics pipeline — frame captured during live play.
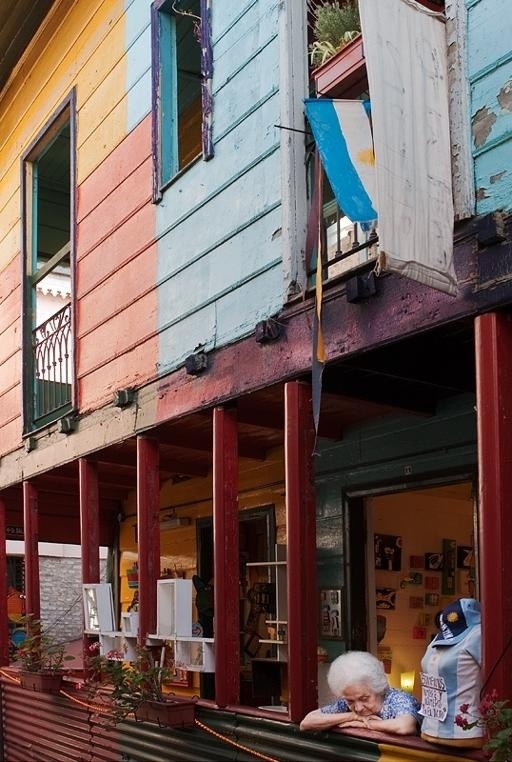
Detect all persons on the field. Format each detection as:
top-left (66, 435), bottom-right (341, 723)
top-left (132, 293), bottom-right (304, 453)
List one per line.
top-left (416, 597), bottom-right (486, 749)
top-left (299, 650), bottom-right (422, 737)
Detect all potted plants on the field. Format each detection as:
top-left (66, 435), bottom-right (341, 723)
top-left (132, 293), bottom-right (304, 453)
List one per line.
top-left (86, 641), bottom-right (195, 761)
top-left (16, 635), bottom-right (66, 692)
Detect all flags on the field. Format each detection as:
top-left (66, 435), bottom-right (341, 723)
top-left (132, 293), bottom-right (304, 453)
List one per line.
top-left (301, 97), bottom-right (379, 222)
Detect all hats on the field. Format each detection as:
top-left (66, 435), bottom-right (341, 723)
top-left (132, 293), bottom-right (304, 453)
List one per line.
top-left (430, 597), bottom-right (482, 648)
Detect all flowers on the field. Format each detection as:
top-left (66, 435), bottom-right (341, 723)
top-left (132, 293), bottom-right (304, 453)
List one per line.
top-left (451, 687), bottom-right (512, 761)
